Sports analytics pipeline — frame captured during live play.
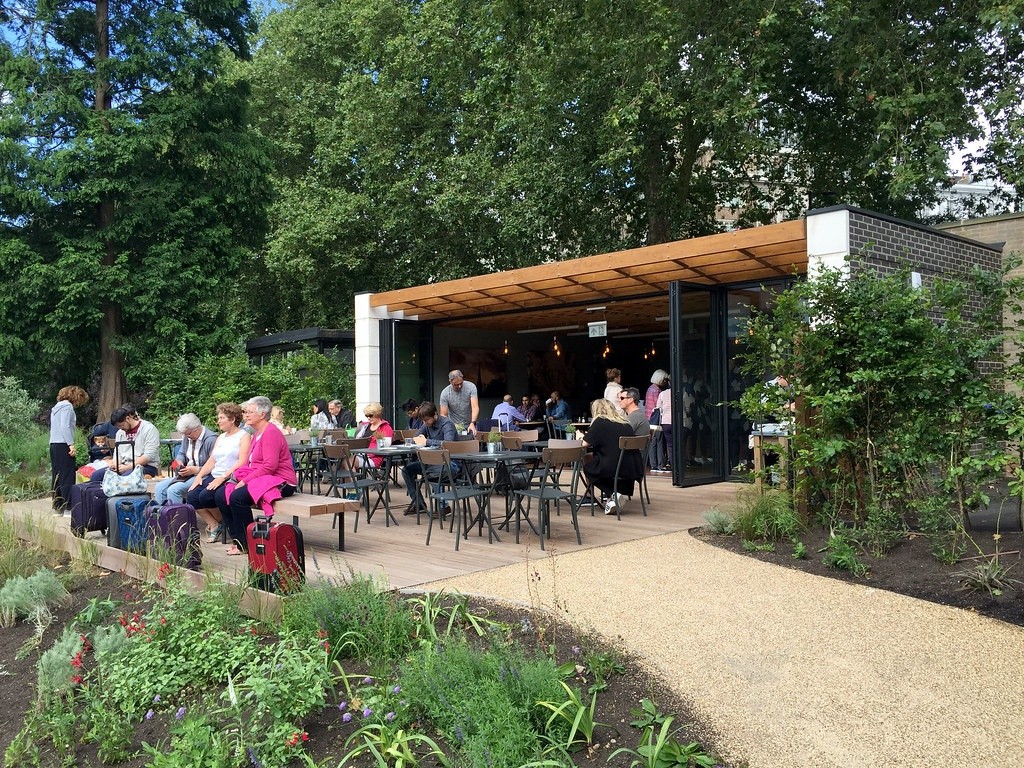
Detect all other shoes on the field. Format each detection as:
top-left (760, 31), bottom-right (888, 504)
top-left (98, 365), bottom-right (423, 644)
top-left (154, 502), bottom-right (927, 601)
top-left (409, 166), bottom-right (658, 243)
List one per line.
top-left (434, 506), bottom-right (452, 517)
top-left (322, 470), bottom-right (328, 484)
top-left (404, 502), bottom-right (426, 515)
top-left (345, 490), bottom-right (364, 501)
top-left (686, 458), bottom-right (713, 468)
top-left (604, 492), bottom-right (627, 515)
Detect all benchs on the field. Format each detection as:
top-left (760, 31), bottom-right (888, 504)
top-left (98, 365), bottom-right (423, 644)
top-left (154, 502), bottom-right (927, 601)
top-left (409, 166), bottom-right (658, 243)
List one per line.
top-left (252, 493), bottom-right (360, 557)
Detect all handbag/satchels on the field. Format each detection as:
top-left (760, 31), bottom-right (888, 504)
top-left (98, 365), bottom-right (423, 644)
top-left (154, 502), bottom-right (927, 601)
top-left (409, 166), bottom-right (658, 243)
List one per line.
top-left (102, 464), bottom-right (148, 497)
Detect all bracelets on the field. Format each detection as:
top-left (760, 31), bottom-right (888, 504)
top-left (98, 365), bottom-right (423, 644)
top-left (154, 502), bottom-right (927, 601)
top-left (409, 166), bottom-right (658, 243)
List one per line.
top-left (223, 473), bottom-right (229, 480)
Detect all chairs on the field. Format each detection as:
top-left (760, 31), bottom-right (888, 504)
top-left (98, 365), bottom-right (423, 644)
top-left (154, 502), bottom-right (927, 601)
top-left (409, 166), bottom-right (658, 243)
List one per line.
top-left (290, 428), bottom-right (655, 551)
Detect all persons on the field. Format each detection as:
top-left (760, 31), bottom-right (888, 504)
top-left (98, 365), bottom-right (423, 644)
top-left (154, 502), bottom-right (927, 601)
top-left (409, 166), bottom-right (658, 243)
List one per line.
top-left (238, 401), bottom-right (257, 435)
top-left (341, 402), bottom-right (394, 501)
top-left (604, 367), bottom-right (628, 418)
top-left (644, 368), bottom-right (674, 473)
top-left (186, 402), bottom-right (252, 543)
top-left (155, 413), bottom-right (219, 506)
top-left (49, 385), bottom-right (89, 517)
top-left (90, 402), bottom-right (160, 483)
top-left (575, 388), bottom-right (651, 506)
top-left (682, 370), bottom-right (713, 468)
top-left (309, 399), bottom-right (355, 484)
top-left (401, 400), bottom-right (462, 518)
top-left (491, 391), bottom-right (573, 426)
top-left (581, 398), bottom-right (644, 515)
top-left (439, 370), bottom-right (480, 437)
top-left (214, 395), bottom-right (299, 556)
top-left (398, 397), bottom-right (425, 430)
top-left (268, 406), bottom-right (291, 435)
top-left (755, 374), bottom-right (795, 424)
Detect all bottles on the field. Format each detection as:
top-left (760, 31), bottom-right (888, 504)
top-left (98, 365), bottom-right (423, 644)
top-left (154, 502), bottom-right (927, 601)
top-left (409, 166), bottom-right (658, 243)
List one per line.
top-left (578, 417), bottom-right (581, 422)
top-left (582, 417), bottom-right (585, 422)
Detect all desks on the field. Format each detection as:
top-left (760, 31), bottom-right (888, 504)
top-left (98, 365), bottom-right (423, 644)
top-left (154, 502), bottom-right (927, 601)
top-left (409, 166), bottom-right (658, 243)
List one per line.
top-left (288, 444), bottom-right (324, 495)
top-left (450, 451), bottom-right (544, 542)
top-left (160, 439), bottom-right (183, 477)
top-left (565, 423), bottom-right (591, 470)
top-left (519, 422), bottom-right (546, 459)
top-left (521, 441), bottom-right (604, 514)
top-left (350, 448), bottom-right (430, 526)
top-left (752, 431), bottom-right (790, 493)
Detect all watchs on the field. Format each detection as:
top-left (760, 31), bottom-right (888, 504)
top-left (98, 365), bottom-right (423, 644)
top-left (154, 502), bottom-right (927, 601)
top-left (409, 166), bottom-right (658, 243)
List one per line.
top-left (470, 421), bottom-right (477, 426)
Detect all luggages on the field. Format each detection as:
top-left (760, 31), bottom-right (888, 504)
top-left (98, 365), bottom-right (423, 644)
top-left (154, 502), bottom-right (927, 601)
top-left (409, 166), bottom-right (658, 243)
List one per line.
top-left (146, 499), bottom-right (202, 569)
top-left (246, 515), bottom-right (305, 597)
top-left (71, 480), bottom-right (108, 539)
top-left (105, 441), bottom-right (151, 556)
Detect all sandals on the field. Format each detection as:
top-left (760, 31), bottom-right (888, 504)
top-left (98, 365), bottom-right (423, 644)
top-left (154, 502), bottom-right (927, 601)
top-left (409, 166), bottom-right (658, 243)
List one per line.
top-left (206, 524), bottom-right (222, 543)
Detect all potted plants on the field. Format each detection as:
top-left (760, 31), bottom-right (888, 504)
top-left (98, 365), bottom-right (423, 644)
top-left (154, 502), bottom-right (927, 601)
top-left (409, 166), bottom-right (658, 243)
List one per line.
top-left (308, 428), bottom-right (319, 446)
top-left (372, 427), bottom-right (386, 450)
top-left (454, 422), bottom-right (465, 435)
top-left (487, 431), bottom-right (503, 454)
top-left (565, 423), bottom-right (577, 440)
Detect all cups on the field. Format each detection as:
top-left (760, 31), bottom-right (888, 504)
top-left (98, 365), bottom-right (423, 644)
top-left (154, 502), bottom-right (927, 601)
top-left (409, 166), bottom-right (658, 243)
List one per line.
top-left (566, 433), bottom-right (572, 440)
top-left (325, 435), bottom-right (332, 445)
top-left (405, 438), bottom-right (411, 447)
top-left (546, 398), bottom-right (552, 404)
top-left (497, 442), bottom-right (502, 451)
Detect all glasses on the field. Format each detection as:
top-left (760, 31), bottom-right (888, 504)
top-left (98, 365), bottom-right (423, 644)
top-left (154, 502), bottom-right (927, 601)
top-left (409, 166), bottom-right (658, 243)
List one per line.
top-left (116, 420), bottom-right (125, 430)
top-left (365, 414), bottom-right (373, 418)
top-left (618, 397), bottom-right (631, 400)
top-left (404, 411), bottom-right (413, 415)
top-left (242, 410), bottom-right (247, 413)
top-left (182, 430), bottom-right (192, 438)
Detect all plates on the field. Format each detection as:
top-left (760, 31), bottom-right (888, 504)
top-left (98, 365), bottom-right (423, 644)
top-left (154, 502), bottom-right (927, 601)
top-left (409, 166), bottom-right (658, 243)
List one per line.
top-left (396, 445), bottom-right (417, 449)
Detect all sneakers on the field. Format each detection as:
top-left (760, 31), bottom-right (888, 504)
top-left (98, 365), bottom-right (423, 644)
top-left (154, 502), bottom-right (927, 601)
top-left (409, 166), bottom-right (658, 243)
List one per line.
top-left (599, 493), bottom-right (608, 500)
top-left (650, 464), bottom-right (673, 473)
top-left (575, 496), bottom-right (598, 506)
